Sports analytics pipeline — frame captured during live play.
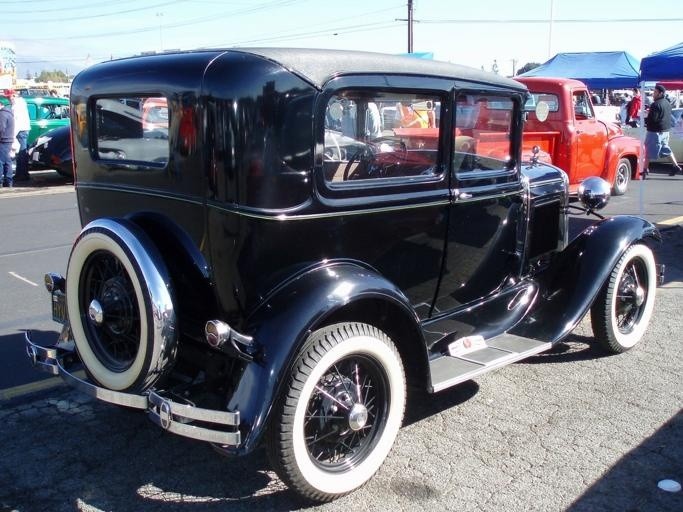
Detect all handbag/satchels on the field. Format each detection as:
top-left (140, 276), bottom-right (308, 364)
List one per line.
top-left (626, 121), bottom-right (638, 127)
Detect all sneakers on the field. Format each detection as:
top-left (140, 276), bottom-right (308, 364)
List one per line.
top-left (641, 169), bottom-right (649, 175)
top-left (669, 165), bottom-right (682, 176)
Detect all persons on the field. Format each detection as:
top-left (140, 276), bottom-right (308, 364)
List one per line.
top-left (638, 84), bottom-right (682, 177)
top-left (0, 95), bottom-right (14, 190)
top-left (608, 87), bottom-right (652, 129)
top-left (324, 95), bottom-right (436, 146)
top-left (2, 88), bottom-right (31, 182)
top-left (48, 89), bottom-right (69, 119)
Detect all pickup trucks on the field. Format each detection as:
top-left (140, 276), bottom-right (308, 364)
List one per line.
top-left (393, 77), bottom-right (647, 201)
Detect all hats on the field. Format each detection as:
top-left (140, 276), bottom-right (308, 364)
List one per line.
top-left (655, 86), bottom-right (665, 92)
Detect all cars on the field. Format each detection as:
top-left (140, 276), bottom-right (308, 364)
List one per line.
top-left (31, 99), bottom-right (172, 180)
top-left (0, 94), bottom-right (73, 147)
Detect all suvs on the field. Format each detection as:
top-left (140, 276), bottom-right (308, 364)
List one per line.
top-left (22, 44), bottom-right (667, 505)
